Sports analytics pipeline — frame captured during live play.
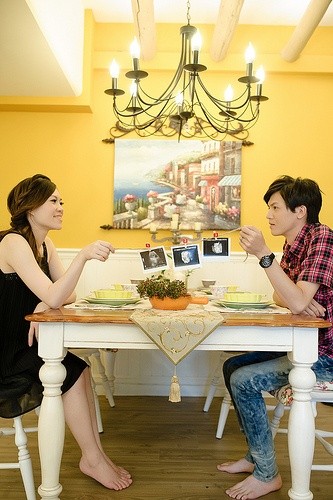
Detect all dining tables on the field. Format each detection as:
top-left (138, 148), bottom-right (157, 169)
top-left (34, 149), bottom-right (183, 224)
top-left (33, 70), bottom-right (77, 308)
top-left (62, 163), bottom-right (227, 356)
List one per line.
top-left (24, 291), bottom-right (333, 500)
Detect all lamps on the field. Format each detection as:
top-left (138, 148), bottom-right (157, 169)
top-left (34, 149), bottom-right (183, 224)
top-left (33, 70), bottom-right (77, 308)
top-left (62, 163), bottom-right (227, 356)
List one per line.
top-left (104, 0), bottom-right (269, 142)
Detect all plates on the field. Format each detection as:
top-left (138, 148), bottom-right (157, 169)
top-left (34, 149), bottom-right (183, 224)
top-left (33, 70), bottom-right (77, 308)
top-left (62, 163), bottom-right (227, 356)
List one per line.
top-left (85, 297), bottom-right (140, 306)
top-left (217, 299), bottom-right (275, 309)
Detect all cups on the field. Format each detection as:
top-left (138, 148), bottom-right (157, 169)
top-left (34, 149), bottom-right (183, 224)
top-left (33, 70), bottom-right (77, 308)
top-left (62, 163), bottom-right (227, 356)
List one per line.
top-left (131, 280), bottom-right (144, 285)
top-left (202, 281), bottom-right (216, 288)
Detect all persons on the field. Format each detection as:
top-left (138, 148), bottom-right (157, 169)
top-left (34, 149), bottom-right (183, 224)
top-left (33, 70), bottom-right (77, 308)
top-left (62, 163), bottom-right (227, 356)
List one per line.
top-left (0, 173), bottom-right (133, 490)
top-left (216, 176), bottom-right (333, 500)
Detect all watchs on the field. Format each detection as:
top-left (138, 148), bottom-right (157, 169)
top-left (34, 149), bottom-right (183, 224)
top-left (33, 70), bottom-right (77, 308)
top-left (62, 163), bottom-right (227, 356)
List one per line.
top-left (259, 253), bottom-right (275, 268)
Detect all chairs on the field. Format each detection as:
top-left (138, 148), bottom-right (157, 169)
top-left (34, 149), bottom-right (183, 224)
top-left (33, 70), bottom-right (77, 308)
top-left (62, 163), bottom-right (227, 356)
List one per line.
top-left (0, 300), bottom-right (333, 500)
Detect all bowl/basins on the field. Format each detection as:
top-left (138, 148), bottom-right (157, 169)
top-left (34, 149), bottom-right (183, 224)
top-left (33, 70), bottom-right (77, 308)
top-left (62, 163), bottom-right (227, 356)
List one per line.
top-left (98, 289), bottom-right (134, 298)
top-left (228, 287), bottom-right (237, 292)
top-left (115, 285), bottom-right (122, 290)
top-left (225, 293), bottom-right (262, 301)
